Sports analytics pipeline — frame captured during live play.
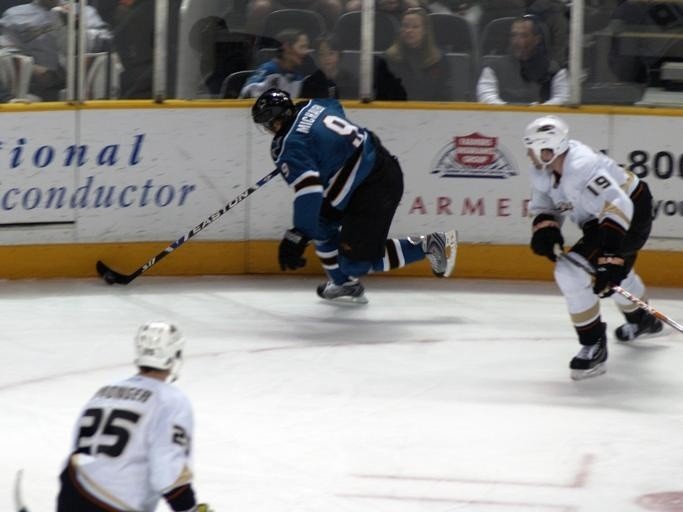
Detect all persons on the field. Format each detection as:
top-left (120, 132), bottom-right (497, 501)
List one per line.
top-left (1, 1), bottom-right (181, 105)
top-left (250, 88), bottom-right (459, 304)
top-left (234, 2), bottom-right (682, 106)
top-left (523, 115), bottom-right (664, 379)
top-left (55, 318), bottom-right (215, 511)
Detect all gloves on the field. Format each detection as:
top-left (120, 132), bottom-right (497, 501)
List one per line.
top-left (530, 212), bottom-right (564, 262)
top-left (279, 227), bottom-right (311, 271)
top-left (594, 256), bottom-right (625, 298)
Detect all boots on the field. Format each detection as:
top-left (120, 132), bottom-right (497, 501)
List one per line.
top-left (617, 303), bottom-right (662, 341)
top-left (407, 232), bottom-right (447, 278)
top-left (318, 277), bottom-right (364, 298)
top-left (571, 317), bottom-right (606, 369)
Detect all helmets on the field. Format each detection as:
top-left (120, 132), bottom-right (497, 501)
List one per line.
top-left (523, 116), bottom-right (569, 172)
top-left (253, 88), bottom-right (294, 136)
top-left (134, 321), bottom-right (184, 370)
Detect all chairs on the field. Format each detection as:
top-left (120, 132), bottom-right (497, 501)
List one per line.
top-left (216, 9), bottom-right (547, 98)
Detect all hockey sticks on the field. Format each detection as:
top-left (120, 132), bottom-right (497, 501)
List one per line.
top-left (96, 168), bottom-right (280, 285)
